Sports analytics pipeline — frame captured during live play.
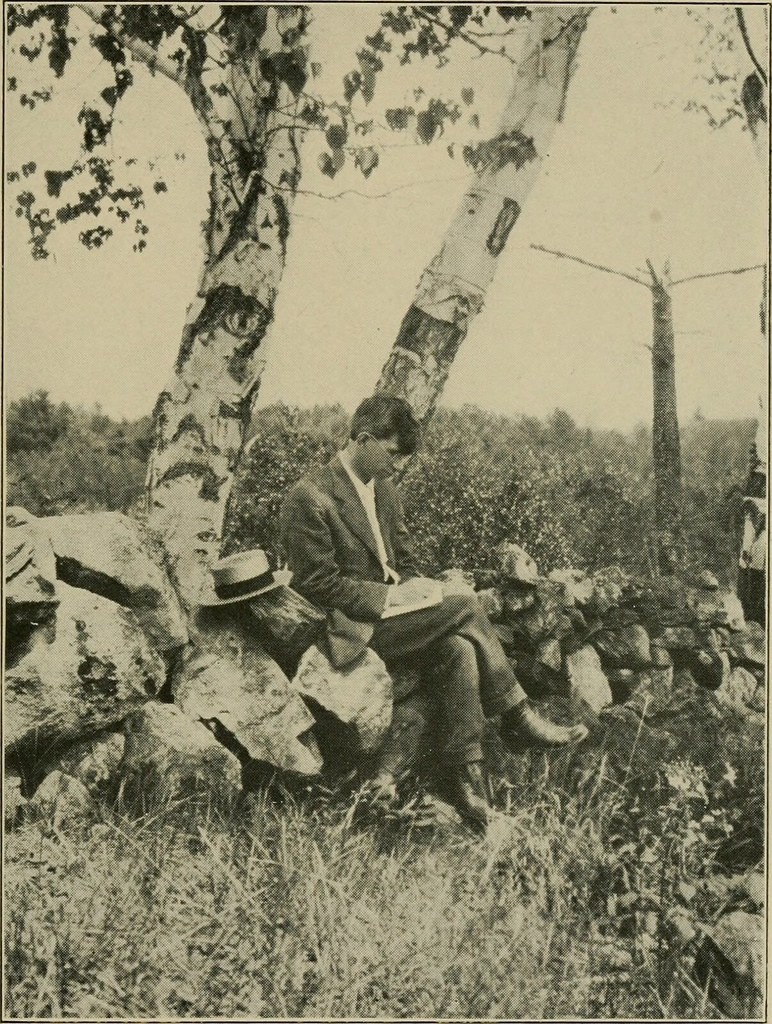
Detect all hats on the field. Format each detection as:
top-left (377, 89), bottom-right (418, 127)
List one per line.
top-left (195, 550), bottom-right (294, 607)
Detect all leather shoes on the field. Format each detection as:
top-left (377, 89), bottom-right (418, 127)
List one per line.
top-left (498, 705), bottom-right (588, 751)
top-left (438, 766), bottom-right (490, 821)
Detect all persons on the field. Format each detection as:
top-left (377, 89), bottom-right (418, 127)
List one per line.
top-left (279, 392), bottom-right (589, 824)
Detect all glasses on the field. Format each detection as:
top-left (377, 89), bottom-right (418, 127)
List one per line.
top-left (358, 431), bottom-right (411, 467)
